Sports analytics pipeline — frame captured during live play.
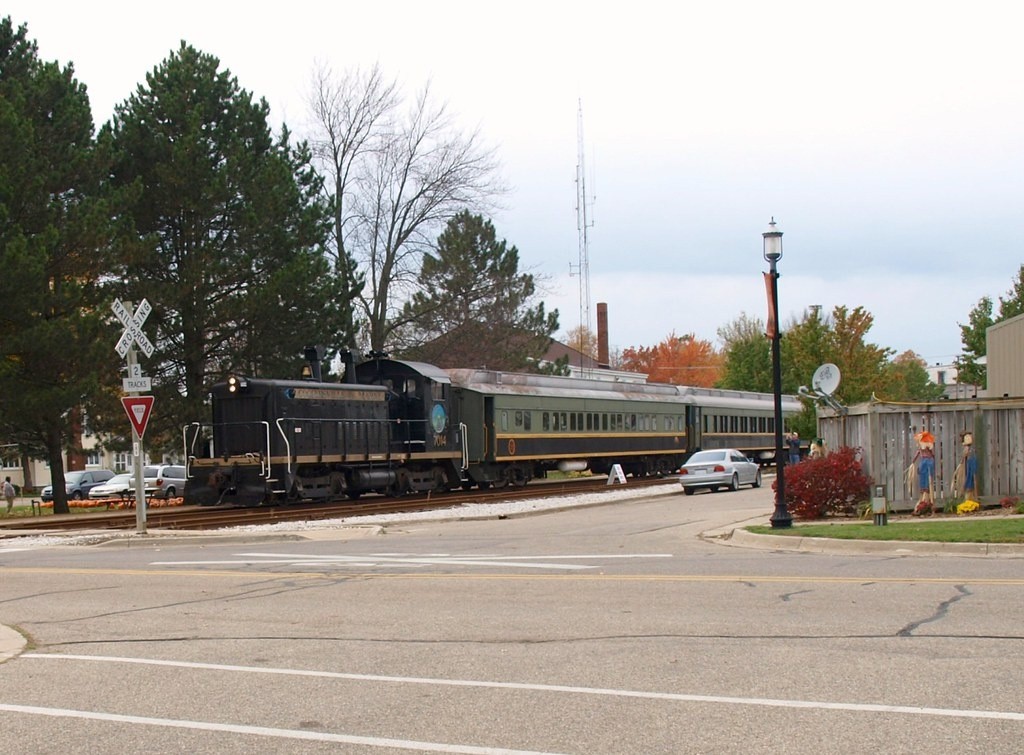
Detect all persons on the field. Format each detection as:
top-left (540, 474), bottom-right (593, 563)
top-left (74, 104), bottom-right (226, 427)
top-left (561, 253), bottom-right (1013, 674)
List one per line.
top-left (786, 432), bottom-right (800, 464)
top-left (3, 476), bottom-right (16, 515)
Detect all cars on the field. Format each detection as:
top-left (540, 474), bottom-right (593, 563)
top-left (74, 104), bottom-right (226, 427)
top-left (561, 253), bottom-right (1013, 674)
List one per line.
top-left (679, 449), bottom-right (762, 496)
top-left (88, 474), bottom-right (134, 499)
top-left (40, 470), bottom-right (116, 502)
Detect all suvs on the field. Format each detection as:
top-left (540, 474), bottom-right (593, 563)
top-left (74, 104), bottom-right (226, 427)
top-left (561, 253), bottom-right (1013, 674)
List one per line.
top-left (128, 463), bottom-right (188, 500)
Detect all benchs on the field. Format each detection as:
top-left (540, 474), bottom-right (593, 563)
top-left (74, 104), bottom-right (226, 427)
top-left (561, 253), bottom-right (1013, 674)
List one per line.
top-left (100, 497), bottom-right (135, 510)
top-left (119, 495), bottom-right (156, 499)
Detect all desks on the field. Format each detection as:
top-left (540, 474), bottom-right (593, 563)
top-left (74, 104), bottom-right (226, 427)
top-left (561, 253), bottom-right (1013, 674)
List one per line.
top-left (111, 486), bottom-right (161, 509)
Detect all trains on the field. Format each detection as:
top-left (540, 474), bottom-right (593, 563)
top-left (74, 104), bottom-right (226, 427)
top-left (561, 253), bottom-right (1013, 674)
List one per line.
top-left (182, 347), bottom-right (811, 507)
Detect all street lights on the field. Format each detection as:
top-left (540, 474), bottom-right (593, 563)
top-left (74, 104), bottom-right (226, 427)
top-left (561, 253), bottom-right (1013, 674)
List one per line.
top-left (762, 216), bottom-right (794, 529)
